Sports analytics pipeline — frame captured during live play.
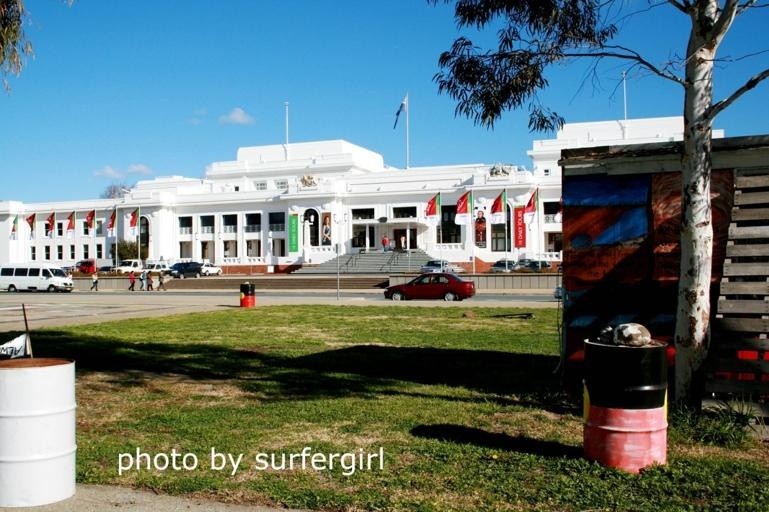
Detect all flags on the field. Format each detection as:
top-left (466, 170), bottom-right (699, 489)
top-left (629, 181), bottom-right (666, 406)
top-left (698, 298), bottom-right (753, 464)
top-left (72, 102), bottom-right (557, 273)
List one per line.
top-left (393, 93), bottom-right (408, 129)
top-left (426, 192), bottom-right (442, 215)
top-left (455, 189), bottom-right (474, 214)
top-left (67, 210), bottom-right (76, 231)
top-left (106, 209), bottom-right (116, 229)
top-left (130, 208), bottom-right (140, 227)
top-left (11, 212), bottom-right (56, 233)
top-left (525, 188), bottom-right (539, 213)
top-left (490, 189), bottom-right (507, 214)
top-left (87, 209), bottom-right (96, 227)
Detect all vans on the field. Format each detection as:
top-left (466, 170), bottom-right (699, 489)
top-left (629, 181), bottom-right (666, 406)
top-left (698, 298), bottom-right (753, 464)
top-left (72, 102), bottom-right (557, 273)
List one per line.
top-left (0, 264), bottom-right (74, 292)
top-left (139, 262), bottom-right (169, 273)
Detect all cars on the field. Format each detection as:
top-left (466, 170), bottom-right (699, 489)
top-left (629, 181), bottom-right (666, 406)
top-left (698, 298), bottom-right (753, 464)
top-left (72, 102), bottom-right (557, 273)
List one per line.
top-left (382, 272), bottom-right (476, 301)
top-left (198, 262), bottom-right (223, 276)
top-left (554, 283), bottom-right (564, 299)
top-left (490, 256), bottom-right (562, 274)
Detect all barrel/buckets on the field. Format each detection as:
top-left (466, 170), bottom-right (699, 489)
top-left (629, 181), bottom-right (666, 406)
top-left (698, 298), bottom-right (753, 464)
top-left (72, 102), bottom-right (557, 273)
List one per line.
top-left (0, 355), bottom-right (76, 509)
top-left (240, 283), bottom-right (256, 307)
top-left (581, 337), bottom-right (671, 475)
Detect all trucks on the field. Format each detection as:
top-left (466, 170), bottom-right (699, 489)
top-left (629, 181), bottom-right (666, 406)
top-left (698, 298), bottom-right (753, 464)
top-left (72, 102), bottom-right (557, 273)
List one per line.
top-left (110, 259), bottom-right (143, 274)
top-left (75, 258), bottom-right (114, 272)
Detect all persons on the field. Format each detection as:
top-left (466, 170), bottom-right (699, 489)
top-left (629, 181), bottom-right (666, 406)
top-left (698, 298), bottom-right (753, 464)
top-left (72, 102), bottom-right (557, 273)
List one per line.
top-left (157, 270), bottom-right (166, 291)
top-left (128, 271), bottom-right (136, 291)
top-left (476, 211), bottom-right (485, 223)
top-left (382, 235), bottom-right (390, 251)
top-left (322, 216), bottom-right (331, 245)
top-left (140, 271), bottom-right (154, 291)
top-left (90, 271), bottom-right (99, 291)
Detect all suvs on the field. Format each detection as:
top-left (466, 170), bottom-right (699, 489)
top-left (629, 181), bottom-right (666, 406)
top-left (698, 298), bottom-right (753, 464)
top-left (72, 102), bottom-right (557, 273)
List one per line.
top-left (419, 259), bottom-right (454, 273)
top-left (164, 261), bottom-right (203, 279)
